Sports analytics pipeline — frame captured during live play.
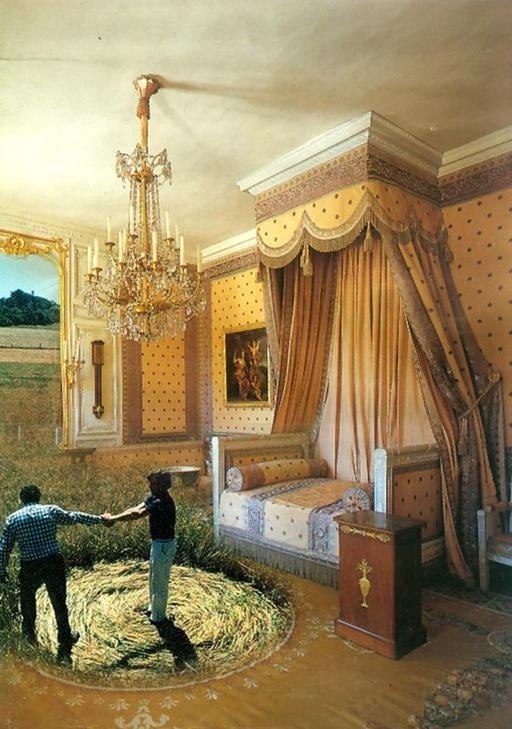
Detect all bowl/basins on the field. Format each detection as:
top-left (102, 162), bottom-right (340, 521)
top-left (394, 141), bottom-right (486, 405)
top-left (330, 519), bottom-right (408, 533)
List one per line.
top-left (160, 466), bottom-right (201, 488)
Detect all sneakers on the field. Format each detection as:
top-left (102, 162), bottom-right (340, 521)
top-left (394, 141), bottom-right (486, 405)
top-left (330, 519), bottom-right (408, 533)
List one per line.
top-left (141, 610), bottom-right (166, 625)
top-left (61, 631), bottom-right (79, 647)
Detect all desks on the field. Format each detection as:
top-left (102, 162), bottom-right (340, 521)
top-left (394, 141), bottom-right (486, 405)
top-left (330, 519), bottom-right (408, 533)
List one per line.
top-left (330, 514), bottom-right (430, 658)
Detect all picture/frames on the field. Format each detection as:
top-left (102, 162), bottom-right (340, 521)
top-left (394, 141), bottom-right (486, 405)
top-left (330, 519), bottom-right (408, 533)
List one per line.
top-left (219, 320), bottom-right (272, 409)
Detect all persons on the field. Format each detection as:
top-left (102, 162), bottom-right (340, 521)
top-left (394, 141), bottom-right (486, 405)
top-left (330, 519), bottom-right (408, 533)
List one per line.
top-left (0, 484), bottom-right (114, 643)
top-left (101, 471), bottom-right (178, 625)
top-left (233, 341), bottom-right (266, 402)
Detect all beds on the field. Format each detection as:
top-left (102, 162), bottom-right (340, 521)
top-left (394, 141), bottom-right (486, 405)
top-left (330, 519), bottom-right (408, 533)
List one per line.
top-left (211, 430), bottom-right (448, 594)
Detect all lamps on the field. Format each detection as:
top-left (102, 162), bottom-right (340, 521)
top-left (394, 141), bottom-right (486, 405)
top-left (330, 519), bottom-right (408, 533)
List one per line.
top-left (79, 74), bottom-right (205, 345)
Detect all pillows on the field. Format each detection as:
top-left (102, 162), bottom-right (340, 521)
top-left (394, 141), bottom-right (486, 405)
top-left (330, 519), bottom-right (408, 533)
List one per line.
top-left (224, 458), bottom-right (331, 492)
top-left (341, 484), bottom-right (373, 515)
